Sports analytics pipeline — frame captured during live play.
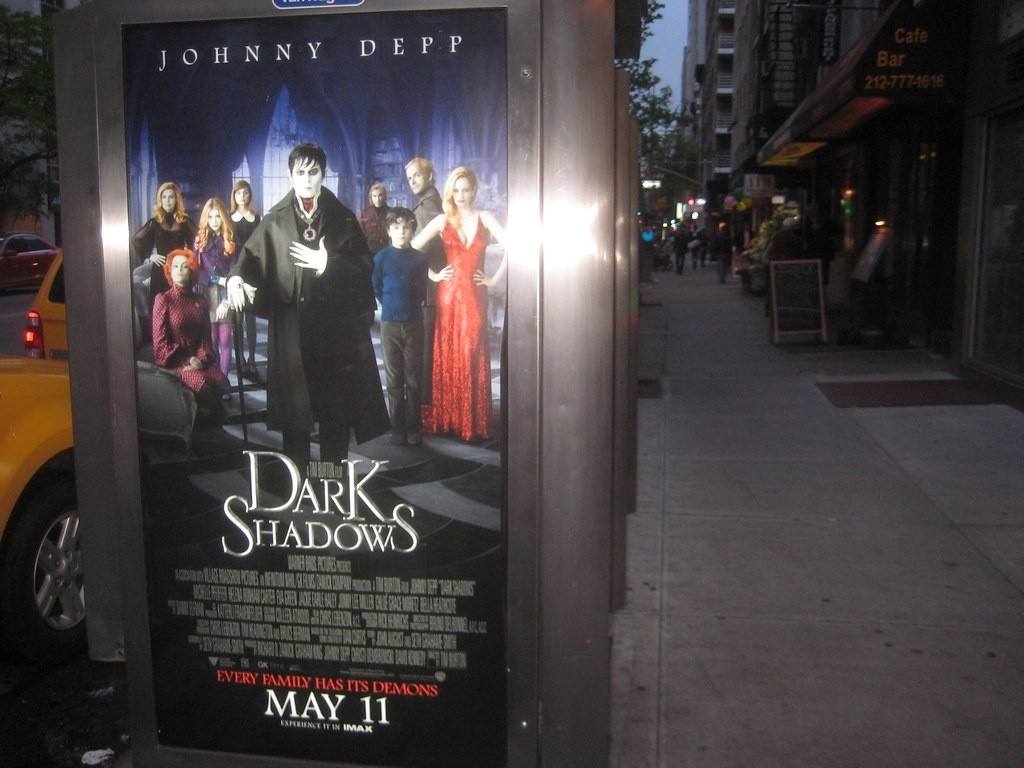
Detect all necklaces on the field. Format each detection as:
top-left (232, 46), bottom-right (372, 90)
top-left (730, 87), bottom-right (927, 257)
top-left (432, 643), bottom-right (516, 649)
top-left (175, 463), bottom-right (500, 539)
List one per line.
top-left (294, 205), bottom-right (322, 242)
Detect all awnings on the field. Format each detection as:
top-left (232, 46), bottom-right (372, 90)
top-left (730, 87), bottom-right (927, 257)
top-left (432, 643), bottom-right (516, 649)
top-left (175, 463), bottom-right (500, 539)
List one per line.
top-left (757, 0), bottom-right (974, 169)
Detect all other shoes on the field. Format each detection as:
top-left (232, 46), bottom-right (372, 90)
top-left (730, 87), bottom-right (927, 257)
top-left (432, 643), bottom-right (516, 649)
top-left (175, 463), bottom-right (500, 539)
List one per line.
top-left (392, 431), bottom-right (406, 445)
top-left (408, 432), bottom-right (422, 445)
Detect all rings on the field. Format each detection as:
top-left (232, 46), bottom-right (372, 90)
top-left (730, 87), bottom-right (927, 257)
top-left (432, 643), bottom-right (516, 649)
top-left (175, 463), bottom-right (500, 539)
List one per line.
top-left (237, 283), bottom-right (241, 288)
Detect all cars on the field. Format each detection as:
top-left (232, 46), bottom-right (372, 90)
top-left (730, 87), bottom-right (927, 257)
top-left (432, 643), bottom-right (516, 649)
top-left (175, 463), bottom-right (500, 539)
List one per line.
top-left (21, 246), bottom-right (67, 359)
top-left (1, 352), bottom-right (90, 647)
top-left (0, 229), bottom-right (61, 290)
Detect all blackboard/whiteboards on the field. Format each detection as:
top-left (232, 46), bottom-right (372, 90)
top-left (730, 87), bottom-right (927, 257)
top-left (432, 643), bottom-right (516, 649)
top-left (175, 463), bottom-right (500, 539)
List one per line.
top-left (769, 260), bottom-right (826, 337)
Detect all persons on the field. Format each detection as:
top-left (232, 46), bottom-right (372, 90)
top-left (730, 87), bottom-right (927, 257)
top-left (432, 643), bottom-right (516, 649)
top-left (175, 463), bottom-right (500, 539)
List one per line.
top-left (196, 197), bottom-right (241, 402)
top-left (226, 142), bottom-right (393, 511)
top-left (689, 225), bottom-right (699, 269)
top-left (358, 183), bottom-right (398, 325)
top-left (372, 206), bottom-right (429, 446)
top-left (410, 166), bottom-right (509, 442)
top-left (224, 181), bottom-right (264, 379)
top-left (698, 228), bottom-right (709, 268)
top-left (404, 157), bottom-right (447, 406)
top-left (712, 221), bottom-right (734, 282)
top-left (132, 181), bottom-right (197, 305)
top-left (153, 249), bottom-right (231, 465)
top-left (673, 228), bottom-right (688, 273)
